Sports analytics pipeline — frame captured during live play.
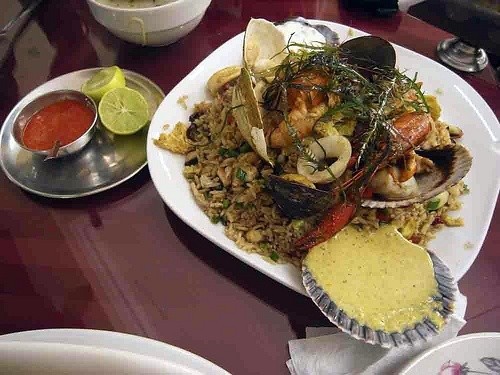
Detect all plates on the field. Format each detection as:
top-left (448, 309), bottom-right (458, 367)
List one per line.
top-left (394, 332), bottom-right (500, 375)
top-left (0, 67), bottom-right (166, 199)
top-left (0, 329), bottom-right (234, 375)
top-left (146, 18), bottom-right (500, 299)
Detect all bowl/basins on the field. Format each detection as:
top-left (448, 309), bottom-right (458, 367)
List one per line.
top-left (86, 0), bottom-right (212, 47)
top-left (11, 89), bottom-right (99, 160)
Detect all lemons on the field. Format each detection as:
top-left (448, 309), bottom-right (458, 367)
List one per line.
top-left (81, 65), bottom-right (126, 104)
top-left (98, 87), bottom-right (149, 135)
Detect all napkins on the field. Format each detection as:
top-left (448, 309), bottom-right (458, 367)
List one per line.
top-left (285, 293), bottom-right (469, 375)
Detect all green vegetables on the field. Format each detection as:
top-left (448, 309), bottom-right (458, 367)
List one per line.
top-left (184, 33), bottom-right (432, 207)
